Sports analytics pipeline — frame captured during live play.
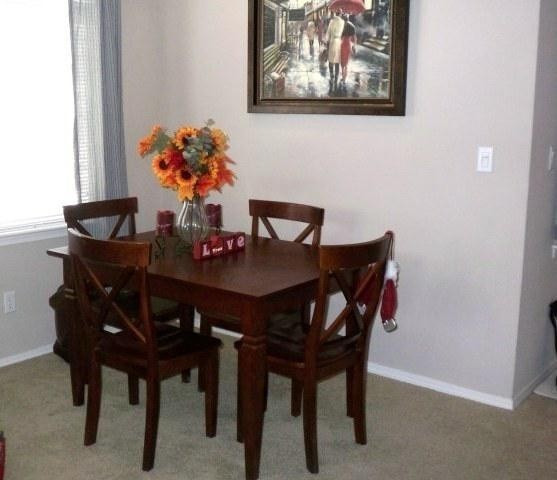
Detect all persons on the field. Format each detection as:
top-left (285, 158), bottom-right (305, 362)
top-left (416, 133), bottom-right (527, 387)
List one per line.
top-left (317, 20), bottom-right (324, 46)
top-left (340, 10), bottom-right (357, 84)
top-left (324, 8), bottom-right (345, 82)
top-left (307, 20), bottom-right (315, 46)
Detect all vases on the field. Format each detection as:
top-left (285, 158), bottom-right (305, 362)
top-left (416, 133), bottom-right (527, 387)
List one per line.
top-left (176, 198), bottom-right (209, 245)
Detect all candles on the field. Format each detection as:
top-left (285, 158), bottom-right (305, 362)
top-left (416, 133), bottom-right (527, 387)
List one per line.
top-left (155, 209), bottom-right (173, 237)
top-left (206, 203), bottom-right (221, 226)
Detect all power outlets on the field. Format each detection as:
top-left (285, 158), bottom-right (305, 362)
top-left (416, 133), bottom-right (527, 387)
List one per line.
top-left (4, 290), bottom-right (16, 314)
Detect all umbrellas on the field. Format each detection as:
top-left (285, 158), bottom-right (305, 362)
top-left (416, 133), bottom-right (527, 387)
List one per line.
top-left (328, 0), bottom-right (366, 15)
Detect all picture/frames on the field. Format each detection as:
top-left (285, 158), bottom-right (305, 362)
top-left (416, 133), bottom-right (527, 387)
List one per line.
top-left (246, 0), bottom-right (410, 117)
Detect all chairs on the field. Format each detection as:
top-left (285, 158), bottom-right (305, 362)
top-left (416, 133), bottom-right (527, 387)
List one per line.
top-left (196, 199), bottom-right (324, 393)
top-left (235, 232), bottom-right (392, 474)
top-left (63, 196), bottom-right (194, 406)
top-left (68, 228), bottom-right (223, 473)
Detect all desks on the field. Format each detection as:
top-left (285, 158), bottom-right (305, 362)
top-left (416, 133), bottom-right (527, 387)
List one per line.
top-left (47, 222), bottom-right (351, 480)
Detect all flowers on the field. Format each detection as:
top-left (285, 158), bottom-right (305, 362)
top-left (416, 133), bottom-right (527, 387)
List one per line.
top-left (136, 118), bottom-right (238, 201)
